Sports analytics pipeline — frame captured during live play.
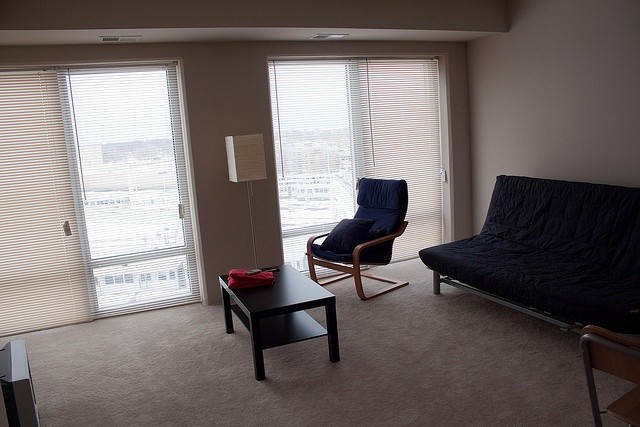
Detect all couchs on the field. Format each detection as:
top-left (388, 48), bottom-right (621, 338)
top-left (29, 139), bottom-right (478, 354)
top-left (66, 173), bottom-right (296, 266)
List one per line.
top-left (418, 174), bottom-right (640, 336)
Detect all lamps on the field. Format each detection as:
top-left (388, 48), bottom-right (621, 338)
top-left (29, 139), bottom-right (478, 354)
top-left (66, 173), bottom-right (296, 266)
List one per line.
top-left (225, 132), bottom-right (268, 269)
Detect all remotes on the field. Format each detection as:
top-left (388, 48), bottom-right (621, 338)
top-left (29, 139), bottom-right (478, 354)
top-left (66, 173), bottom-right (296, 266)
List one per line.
top-left (244, 269), bottom-right (261, 276)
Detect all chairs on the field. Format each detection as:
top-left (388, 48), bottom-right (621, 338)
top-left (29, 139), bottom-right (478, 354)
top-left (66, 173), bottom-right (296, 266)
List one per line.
top-left (304, 177), bottom-right (409, 300)
top-left (578, 323), bottom-right (640, 426)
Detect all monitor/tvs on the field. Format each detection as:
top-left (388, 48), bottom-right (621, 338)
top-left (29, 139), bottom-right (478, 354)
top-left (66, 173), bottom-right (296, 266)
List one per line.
top-left (0, 339), bottom-right (39, 426)
